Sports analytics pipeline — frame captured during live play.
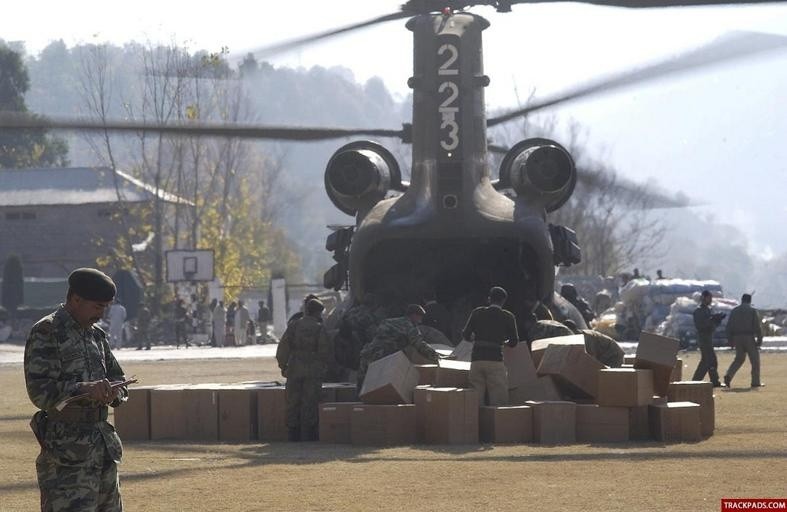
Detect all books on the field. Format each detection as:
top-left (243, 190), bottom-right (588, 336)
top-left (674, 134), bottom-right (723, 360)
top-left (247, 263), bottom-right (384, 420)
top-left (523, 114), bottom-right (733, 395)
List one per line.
top-left (54, 376), bottom-right (141, 413)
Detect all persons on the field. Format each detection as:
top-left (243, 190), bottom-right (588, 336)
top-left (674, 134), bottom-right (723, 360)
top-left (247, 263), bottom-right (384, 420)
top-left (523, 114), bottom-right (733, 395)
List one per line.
top-left (24, 267), bottom-right (130, 512)
top-left (276, 298), bottom-right (332, 441)
top-left (136, 301), bottom-right (151, 350)
top-left (287, 296), bottom-right (324, 326)
top-left (420, 289), bottom-right (455, 341)
top-left (173, 293), bottom-right (272, 349)
top-left (341, 293), bottom-right (379, 341)
top-left (723, 293), bottom-right (766, 386)
top-left (519, 265), bottom-right (673, 404)
top-left (107, 298), bottom-right (127, 350)
top-left (691, 290), bottom-right (727, 387)
top-left (463, 286), bottom-right (518, 406)
top-left (356, 304), bottom-right (457, 401)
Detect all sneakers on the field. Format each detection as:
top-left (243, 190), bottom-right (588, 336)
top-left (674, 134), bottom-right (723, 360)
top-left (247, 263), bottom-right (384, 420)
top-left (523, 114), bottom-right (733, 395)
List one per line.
top-left (724, 376), bottom-right (730, 389)
top-left (750, 383), bottom-right (766, 388)
top-left (712, 385), bottom-right (724, 388)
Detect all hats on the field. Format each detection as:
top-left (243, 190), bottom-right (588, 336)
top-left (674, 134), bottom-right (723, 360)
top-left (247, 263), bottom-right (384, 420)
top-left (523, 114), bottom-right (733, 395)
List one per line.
top-left (308, 299), bottom-right (324, 311)
top-left (407, 303), bottom-right (426, 315)
top-left (66, 266), bottom-right (117, 302)
top-left (489, 286), bottom-right (508, 298)
top-left (560, 283), bottom-right (577, 297)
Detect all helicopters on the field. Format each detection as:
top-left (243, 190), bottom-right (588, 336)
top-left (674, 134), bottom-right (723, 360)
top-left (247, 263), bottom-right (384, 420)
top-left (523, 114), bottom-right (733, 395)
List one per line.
top-left (0, 1), bottom-right (786, 342)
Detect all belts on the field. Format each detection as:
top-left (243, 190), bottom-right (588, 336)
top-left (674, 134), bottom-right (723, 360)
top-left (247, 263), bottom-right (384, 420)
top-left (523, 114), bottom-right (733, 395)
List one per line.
top-left (43, 405), bottom-right (109, 423)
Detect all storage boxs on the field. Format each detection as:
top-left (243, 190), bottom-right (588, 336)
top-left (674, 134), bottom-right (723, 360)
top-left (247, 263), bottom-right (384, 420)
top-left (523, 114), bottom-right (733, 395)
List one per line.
top-left (509, 374), bottom-right (562, 402)
top-left (599, 368), bottom-right (654, 407)
top-left (219, 384), bottom-right (271, 441)
top-left (437, 359), bottom-right (471, 389)
top-left (115, 386), bottom-right (157, 442)
top-left (503, 339), bottom-right (537, 391)
top-left (649, 401), bottom-right (701, 441)
top-left (632, 331), bottom-right (681, 396)
top-left (181, 381), bottom-right (219, 441)
top-left (150, 385), bottom-right (184, 439)
top-left (428, 342), bottom-right (455, 357)
top-left (318, 402), bottom-right (367, 439)
top-left (668, 382), bottom-right (714, 436)
top-left (480, 404), bottom-right (533, 444)
top-left (413, 383), bottom-right (479, 443)
top-left (624, 354), bottom-right (635, 363)
top-left (256, 386), bottom-right (290, 438)
top-left (530, 333), bottom-right (586, 368)
top-left (321, 381), bottom-right (357, 402)
top-left (357, 350), bottom-right (420, 404)
top-left (672, 360), bottom-right (682, 381)
top-left (350, 402), bottom-right (416, 445)
top-left (577, 404), bottom-right (631, 442)
top-left (527, 399), bottom-right (577, 443)
top-left (448, 338), bottom-right (474, 363)
top-left (414, 364), bottom-right (438, 383)
top-left (537, 344), bottom-right (603, 399)
